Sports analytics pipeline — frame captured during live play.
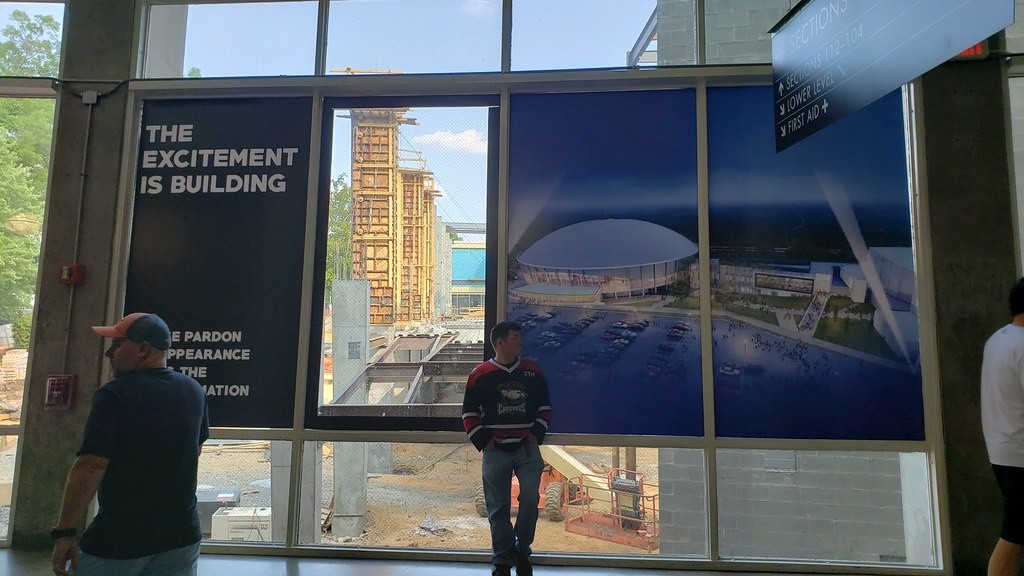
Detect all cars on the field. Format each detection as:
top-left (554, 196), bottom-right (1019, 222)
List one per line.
top-left (511, 309), bottom-right (691, 385)
top-left (719, 366), bottom-right (740, 376)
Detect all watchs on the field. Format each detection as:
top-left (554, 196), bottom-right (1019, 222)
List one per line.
top-left (52, 526), bottom-right (76, 538)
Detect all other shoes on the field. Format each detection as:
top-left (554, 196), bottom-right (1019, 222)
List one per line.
top-left (513, 556), bottom-right (533, 576)
top-left (492, 563), bottom-right (514, 576)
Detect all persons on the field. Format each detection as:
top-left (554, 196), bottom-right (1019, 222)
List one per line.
top-left (52, 312), bottom-right (209, 576)
top-left (509, 279), bottom-right (830, 373)
top-left (463, 323), bottom-right (552, 576)
top-left (981, 276), bottom-right (1024, 576)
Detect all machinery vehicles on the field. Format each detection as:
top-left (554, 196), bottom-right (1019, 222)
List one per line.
top-left (475, 447), bottom-right (593, 522)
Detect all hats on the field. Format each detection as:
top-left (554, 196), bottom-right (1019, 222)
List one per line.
top-left (91, 312), bottom-right (171, 350)
